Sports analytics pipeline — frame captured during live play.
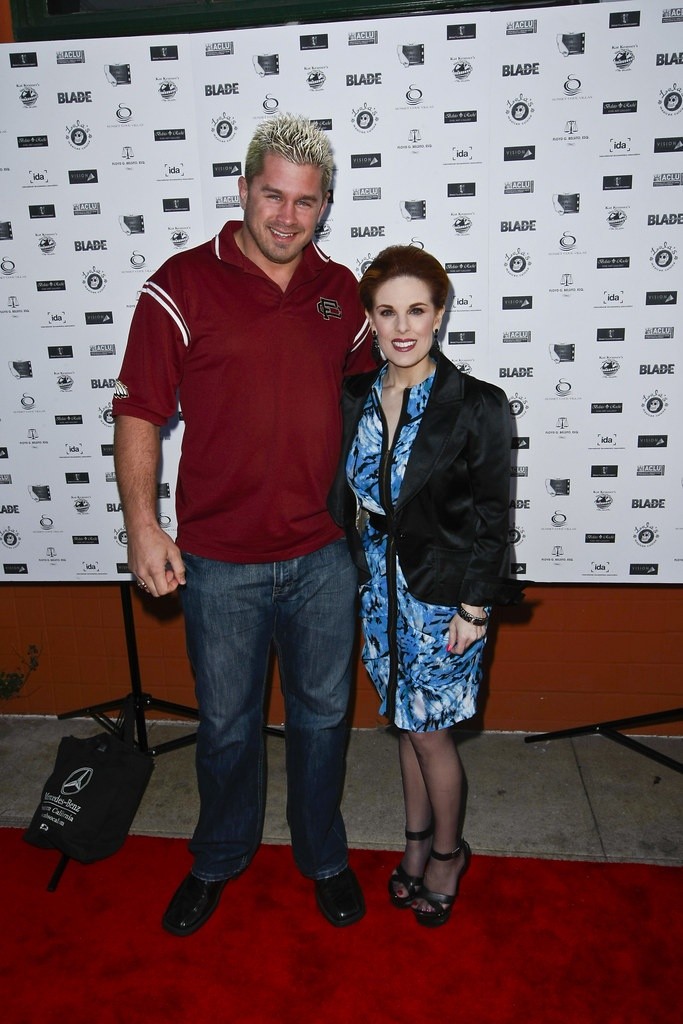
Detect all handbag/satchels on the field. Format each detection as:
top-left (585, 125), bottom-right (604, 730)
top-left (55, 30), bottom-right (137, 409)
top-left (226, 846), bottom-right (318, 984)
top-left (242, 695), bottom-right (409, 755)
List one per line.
top-left (22, 731), bottom-right (154, 865)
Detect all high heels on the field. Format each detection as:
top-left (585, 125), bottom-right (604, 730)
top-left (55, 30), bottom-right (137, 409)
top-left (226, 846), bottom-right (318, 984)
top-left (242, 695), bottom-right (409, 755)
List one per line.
top-left (388, 826), bottom-right (435, 907)
top-left (411, 838), bottom-right (471, 928)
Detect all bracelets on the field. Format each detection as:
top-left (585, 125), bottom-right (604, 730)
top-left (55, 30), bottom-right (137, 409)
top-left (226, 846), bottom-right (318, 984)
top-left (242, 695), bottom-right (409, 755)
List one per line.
top-left (458, 604), bottom-right (488, 626)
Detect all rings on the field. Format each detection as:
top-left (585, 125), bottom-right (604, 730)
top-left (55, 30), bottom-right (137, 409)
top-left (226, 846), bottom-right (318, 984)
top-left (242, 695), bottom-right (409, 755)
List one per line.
top-left (138, 583), bottom-right (147, 590)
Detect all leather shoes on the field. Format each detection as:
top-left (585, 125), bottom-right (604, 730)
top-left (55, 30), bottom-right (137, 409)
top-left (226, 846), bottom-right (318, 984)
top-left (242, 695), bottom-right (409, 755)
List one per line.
top-left (315, 868), bottom-right (366, 926)
top-left (161, 871), bottom-right (243, 936)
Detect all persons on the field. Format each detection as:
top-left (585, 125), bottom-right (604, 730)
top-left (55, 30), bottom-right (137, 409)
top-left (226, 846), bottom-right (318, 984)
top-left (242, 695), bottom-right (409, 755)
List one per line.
top-left (331, 246), bottom-right (513, 925)
top-left (113, 116), bottom-right (380, 934)
